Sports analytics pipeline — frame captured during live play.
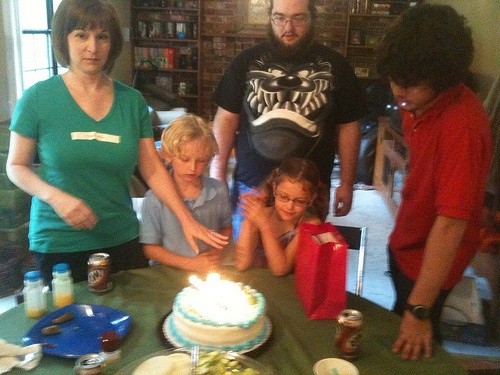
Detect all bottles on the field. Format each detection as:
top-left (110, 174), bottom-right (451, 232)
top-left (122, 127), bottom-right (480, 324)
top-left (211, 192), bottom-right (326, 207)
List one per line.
top-left (22, 271), bottom-right (46, 318)
top-left (52, 263), bottom-right (73, 308)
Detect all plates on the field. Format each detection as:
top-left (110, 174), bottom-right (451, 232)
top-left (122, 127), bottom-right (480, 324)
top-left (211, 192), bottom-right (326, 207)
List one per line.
top-left (25, 303), bottom-right (132, 359)
top-left (312, 355), bottom-right (361, 375)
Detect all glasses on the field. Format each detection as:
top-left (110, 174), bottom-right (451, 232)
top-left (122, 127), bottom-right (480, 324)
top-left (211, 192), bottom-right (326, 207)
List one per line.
top-left (270, 14), bottom-right (313, 24)
top-left (273, 186), bottom-right (313, 208)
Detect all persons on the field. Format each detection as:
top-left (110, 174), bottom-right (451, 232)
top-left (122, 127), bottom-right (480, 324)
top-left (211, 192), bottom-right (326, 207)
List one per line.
top-left (139, 114), bottom-right (233, 273)
top-left (210, 0), bottom-right (365, 217)
top-left (232, 157), bottom-right (323, 277)
top-left (375, 4), bottom-right (492, 362)
top-left (5, 0), bottom-right (230, 292)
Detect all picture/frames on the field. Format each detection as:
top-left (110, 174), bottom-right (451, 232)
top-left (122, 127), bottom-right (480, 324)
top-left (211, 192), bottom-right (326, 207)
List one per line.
top-left (236, 0), bottom-right (272, 36)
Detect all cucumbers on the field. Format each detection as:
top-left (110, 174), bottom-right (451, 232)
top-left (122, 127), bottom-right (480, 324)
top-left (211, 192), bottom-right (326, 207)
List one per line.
top-left (194, 350), bottom-right (262, 375)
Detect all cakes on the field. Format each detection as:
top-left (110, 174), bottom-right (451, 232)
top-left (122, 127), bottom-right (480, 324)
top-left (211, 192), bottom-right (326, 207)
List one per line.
top-left (168, 278), bottom-right (268, 352)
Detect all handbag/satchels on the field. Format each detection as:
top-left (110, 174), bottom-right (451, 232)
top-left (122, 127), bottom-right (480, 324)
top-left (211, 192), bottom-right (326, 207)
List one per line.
top-left (294, 220), bottom-right (348, 321)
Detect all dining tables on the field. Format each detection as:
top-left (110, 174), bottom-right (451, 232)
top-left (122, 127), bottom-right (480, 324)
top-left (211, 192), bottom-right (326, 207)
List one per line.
top-left (0, 261), bottom-right (465, 374)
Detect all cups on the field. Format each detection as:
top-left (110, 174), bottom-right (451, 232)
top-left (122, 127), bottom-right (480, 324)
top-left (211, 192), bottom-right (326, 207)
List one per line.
top-left (175, 22), bottom-right (189, 38)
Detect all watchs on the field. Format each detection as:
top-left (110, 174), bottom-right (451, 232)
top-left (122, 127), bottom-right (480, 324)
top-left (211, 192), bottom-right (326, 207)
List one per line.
top-left (404, 302), bottom-right (435, 319)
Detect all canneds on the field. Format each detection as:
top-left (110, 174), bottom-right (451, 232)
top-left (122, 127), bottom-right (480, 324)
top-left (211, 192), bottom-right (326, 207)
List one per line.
top-left (87, 253), bottom-right (113, 292)
top-left (335, 309), bottom-right (363, 358)
top-left (73, 353), bottom-right (105, 375)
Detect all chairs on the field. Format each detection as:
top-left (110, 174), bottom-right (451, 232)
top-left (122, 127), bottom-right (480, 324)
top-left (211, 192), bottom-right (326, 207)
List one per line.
top-left (331, 224), bottom-right (368, 299)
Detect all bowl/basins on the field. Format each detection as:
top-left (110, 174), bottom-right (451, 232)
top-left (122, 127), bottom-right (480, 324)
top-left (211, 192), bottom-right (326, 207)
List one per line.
top-left (116, 344), bottom-right (274, 374)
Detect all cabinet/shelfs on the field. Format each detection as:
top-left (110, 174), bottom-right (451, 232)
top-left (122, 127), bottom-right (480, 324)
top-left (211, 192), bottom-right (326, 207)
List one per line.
top-left (344, 0), bottom-right (422, 118)
top-left (128, 0), bottom-right (204, 116)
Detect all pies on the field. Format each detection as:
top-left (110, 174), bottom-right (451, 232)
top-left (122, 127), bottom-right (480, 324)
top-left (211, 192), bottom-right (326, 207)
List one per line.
top-left (131, 352), bottom-right (192, 375)
